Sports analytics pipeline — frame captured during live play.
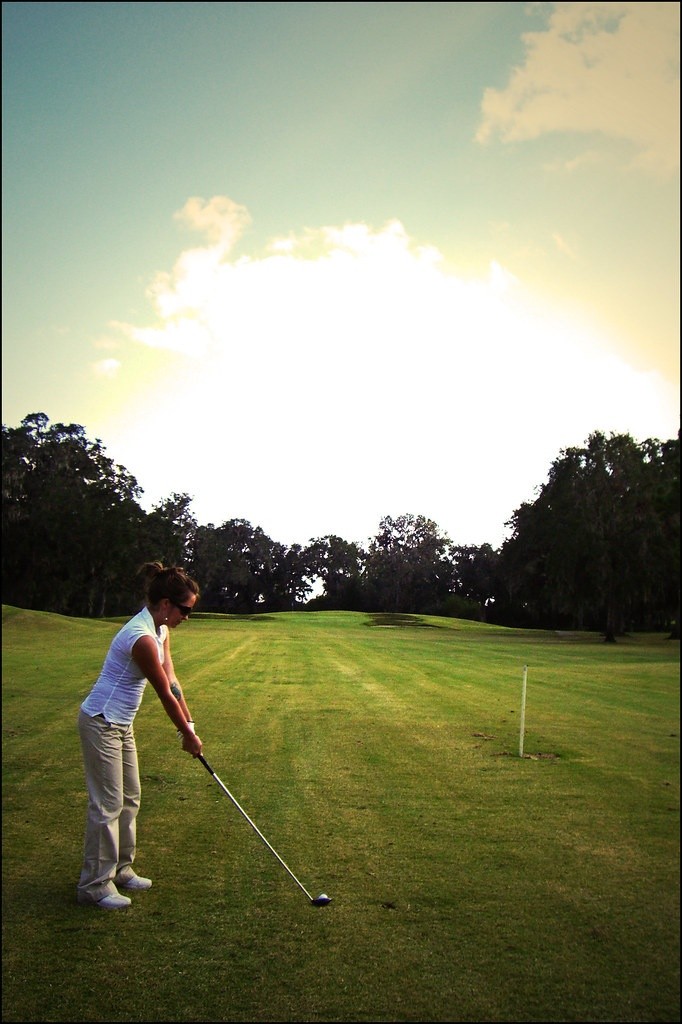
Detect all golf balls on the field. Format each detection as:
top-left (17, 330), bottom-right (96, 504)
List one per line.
top-left (319, 893), bottom-right (328, 898)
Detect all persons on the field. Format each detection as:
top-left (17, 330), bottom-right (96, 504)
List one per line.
top-left (77, 561), bottom-right (203, 911)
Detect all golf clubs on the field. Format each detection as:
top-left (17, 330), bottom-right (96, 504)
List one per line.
top-left (197, 754), bottom-right (333, 907)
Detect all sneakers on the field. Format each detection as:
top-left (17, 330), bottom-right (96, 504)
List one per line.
top-left (96, 892), bottom-right (131, 909)
top-left (123, 874), bottom-right (152, 889)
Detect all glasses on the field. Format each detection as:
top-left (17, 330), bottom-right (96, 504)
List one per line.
top-left (175, 604), bottom-right (193, 616)
